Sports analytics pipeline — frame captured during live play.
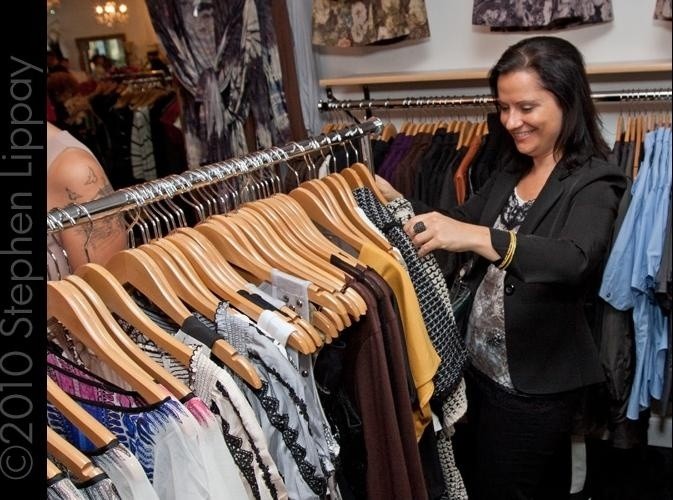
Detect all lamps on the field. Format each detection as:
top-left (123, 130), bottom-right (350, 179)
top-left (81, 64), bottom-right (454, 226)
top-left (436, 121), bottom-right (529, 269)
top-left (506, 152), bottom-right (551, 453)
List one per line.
top-left (94, 1), bottom-right (130, 30)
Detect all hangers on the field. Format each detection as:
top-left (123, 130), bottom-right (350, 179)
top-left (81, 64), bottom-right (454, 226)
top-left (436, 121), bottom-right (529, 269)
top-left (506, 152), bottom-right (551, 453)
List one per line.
top-left (317, 86), bottom-right (499, 150)
top-left (611, 86), bottom-right (672, 180)
top-left (44, 122), bottom-right (391, 482)
top-left (96, 69), bottom-right (165, 112)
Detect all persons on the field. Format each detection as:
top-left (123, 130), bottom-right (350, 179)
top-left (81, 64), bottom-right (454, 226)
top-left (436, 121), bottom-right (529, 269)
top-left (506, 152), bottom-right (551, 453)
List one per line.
top-left (47, 51), bottom-right (171, 128)
top-left (375, 37), bottom-right (626, 500)
top-left (46, 122), bottom-right (128, 281)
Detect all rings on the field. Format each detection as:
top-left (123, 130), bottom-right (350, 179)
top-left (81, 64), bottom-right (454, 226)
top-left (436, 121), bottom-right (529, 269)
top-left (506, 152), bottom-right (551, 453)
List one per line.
top-left (413, 221), bottom-right (426, 233)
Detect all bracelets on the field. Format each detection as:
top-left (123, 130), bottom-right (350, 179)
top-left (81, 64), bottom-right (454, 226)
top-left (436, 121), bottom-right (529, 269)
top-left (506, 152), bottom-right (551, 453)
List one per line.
top-left (497, 230), bottom-right (517, 272)
